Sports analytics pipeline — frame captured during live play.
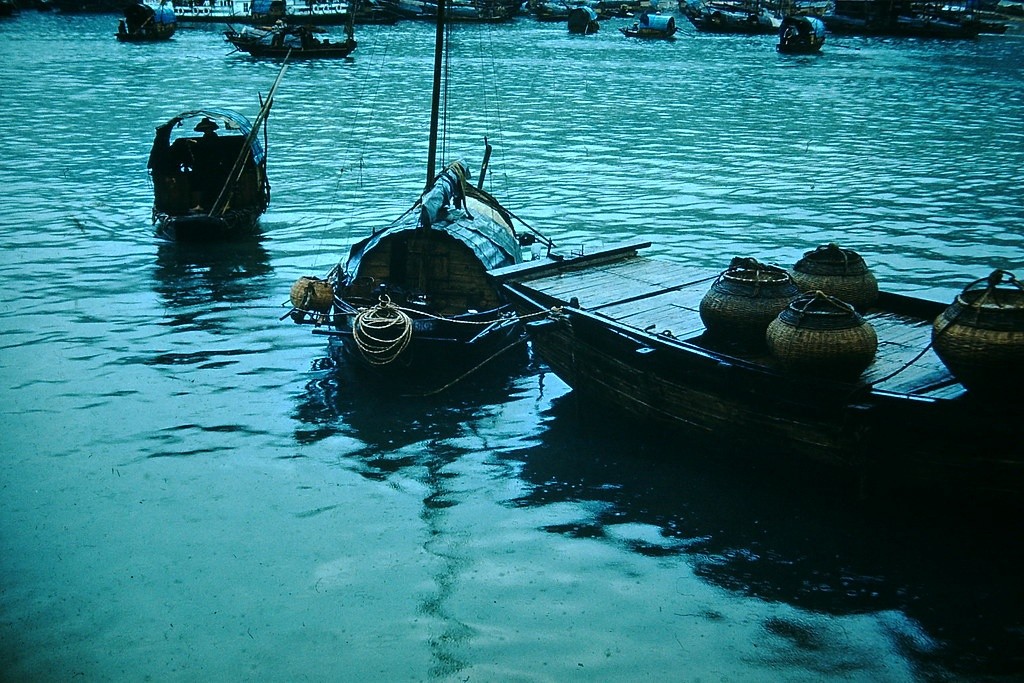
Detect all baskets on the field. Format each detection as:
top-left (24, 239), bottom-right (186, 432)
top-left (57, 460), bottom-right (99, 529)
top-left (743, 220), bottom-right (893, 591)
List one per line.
top-left (290, 277), bottom-right (335, 312)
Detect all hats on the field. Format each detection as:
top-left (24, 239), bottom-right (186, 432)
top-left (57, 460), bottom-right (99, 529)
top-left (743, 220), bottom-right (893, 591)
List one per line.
top-left (195, 118), bottom-right (219, 131)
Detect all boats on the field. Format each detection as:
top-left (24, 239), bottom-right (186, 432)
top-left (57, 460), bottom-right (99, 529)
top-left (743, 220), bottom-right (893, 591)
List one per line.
top-left (142, 106), bottom-right (272, 241)
top-left (279, 0), bottom-right (534, 361)
top-left (112, 3), bottom-right (178, 43)
top-left (484, 228), bottom-right (1023, 483)
top-left (220, 15), bottom-right (359, 58)
top-left (139, 0), bottom-right (828, 54)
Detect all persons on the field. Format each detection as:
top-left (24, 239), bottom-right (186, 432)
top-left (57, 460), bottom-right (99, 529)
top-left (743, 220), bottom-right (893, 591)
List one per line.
top-left (271, 19), bottom-right (284, 46)
top-left (195, 118), bottom-right (219, 142)
